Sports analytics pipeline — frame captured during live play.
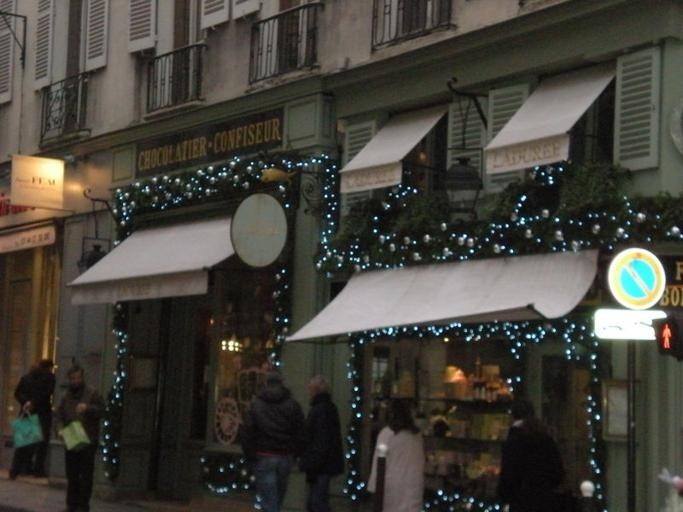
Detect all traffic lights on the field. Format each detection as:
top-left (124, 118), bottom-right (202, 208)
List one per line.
top-left (653, 317), bottom-right (682, 357)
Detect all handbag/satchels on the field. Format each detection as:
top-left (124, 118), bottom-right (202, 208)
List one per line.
top-left (60, 420), bottom-right (92, 452)
top-left (11, 413), bottom-right (44, 448)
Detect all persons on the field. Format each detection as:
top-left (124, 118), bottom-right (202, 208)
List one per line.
top-left (298, 377), bottom-right (344, 512)
top-left (50, 364), bottom-right (105, 512)
top-left (366, 400), bottom-right (425, 512)
top-left (496, 399), bottom-right (564, 512)
top-left (239, 374), bottom-right (305, 512)
top-left (9, 360), bottom-right (55, 480)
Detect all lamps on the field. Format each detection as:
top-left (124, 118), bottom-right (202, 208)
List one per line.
top-left (441, 80), bottom-right (489, 193)
